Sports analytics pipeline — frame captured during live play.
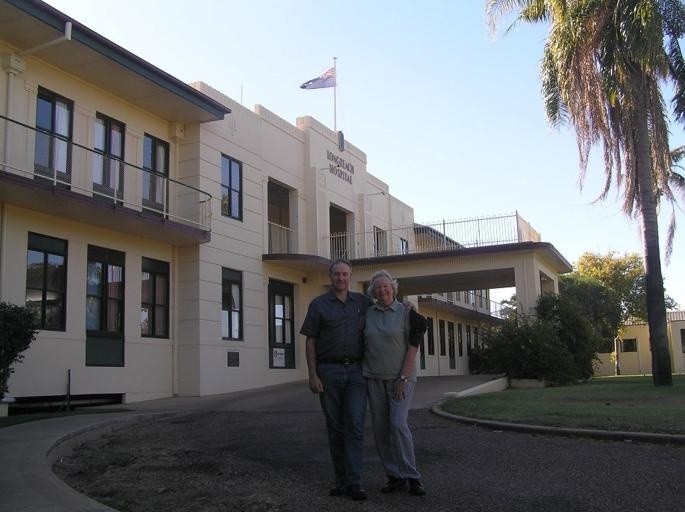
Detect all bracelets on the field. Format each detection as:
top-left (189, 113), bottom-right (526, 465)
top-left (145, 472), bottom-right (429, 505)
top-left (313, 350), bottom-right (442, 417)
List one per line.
top-left (397, 375), bottom-right (408, 383)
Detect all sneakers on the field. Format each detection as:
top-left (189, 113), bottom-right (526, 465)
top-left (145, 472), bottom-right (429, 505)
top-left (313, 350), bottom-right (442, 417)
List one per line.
top-left (329, 481), bottom-right (368, 502)
top-left (382, 477), bottom-right (428, 497)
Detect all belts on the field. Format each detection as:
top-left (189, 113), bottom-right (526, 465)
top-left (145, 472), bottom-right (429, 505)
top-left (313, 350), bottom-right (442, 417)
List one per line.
top-left (322, 358), bottom-right (355, 366)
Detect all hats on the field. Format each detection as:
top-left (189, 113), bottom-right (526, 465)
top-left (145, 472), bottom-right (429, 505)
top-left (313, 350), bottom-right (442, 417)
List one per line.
top-left (408, 308), bottom-right (428, 349)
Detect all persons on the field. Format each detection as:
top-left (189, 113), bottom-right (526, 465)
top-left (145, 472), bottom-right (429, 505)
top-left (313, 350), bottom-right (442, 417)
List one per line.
top-left (300, 258), bottom-right (417, 502)
top-left (361, 269), bottom-right (426, 496)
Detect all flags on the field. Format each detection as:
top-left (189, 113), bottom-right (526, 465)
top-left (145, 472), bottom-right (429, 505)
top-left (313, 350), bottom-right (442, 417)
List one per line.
top-left (299, 68), bottom-right (336, 89)
